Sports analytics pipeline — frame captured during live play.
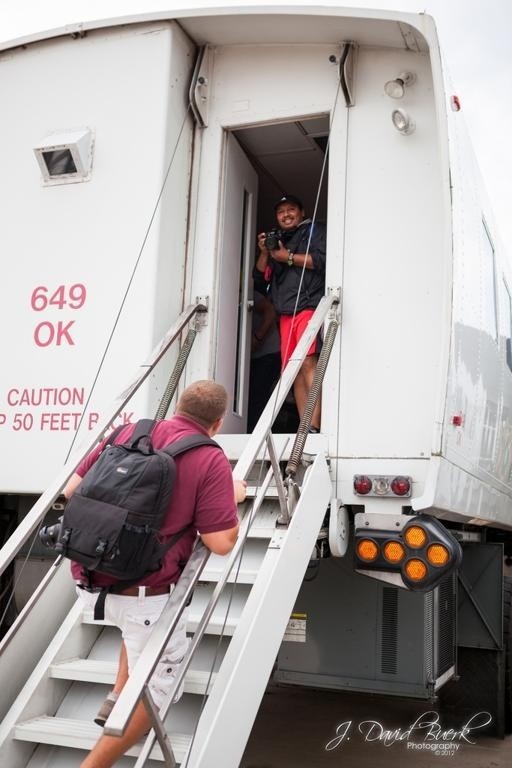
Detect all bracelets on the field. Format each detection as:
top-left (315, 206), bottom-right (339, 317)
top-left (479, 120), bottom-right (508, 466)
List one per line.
top-left (254, 334), bottom-right (262, 342)
top-left (287, 252), bottom-right (293, 266)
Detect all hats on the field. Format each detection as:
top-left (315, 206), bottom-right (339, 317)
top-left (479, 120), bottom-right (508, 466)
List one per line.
top-left (271, 194), bottom-right (304, 211)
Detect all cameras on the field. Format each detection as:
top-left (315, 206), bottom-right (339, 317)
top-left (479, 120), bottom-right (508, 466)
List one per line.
top-left (263, 224), bottom-right (282, 252)
top-left (38, 514), bottom-right (64, 549)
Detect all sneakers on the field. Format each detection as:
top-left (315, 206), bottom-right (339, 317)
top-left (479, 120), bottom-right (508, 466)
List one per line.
top-left (307, 424), bottom-right (319, 434)
top-left (92, 696), bottom-right (117, 730)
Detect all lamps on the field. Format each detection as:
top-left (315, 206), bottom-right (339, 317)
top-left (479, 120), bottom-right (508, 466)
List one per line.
top-left (383, 69), bottom-right (417, 135)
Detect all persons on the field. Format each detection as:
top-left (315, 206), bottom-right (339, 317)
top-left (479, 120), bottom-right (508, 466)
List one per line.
top-left (256, 192), bottom-right (325, 434)
top-left (53, 379), bottom-right (248, 767)
top-left (249, 285), bottom-right (281, 437)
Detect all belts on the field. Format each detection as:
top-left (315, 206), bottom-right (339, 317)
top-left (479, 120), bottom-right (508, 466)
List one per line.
top-left (77, 579), bottom-right (178, 599)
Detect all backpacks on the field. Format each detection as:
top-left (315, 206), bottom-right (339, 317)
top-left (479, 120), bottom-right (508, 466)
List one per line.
top-left (50, 416), bottom-right (224, 583)
